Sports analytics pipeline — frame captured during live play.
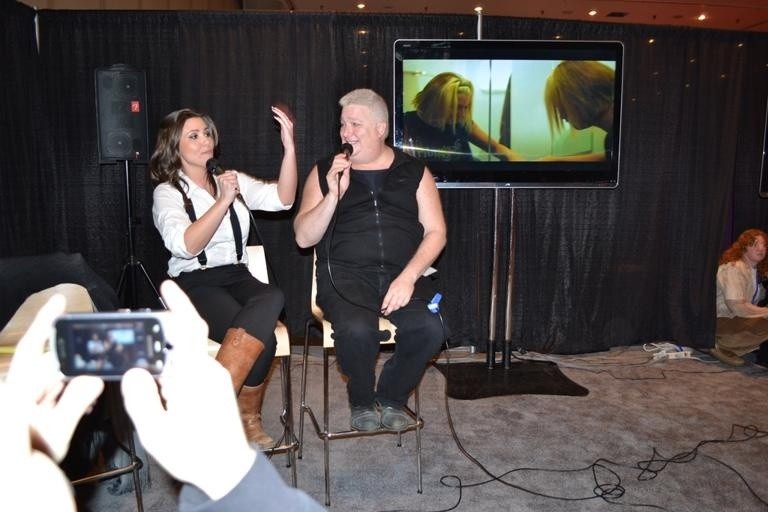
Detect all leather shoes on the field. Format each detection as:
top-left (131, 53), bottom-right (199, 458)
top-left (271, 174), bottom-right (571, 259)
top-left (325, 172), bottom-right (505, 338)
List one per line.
top-left (708, 348), bottom-right (747, 366)
top-left (345, 403), bottom-right (380, 433)
top-left (380, 401), bottom-right (407, 432)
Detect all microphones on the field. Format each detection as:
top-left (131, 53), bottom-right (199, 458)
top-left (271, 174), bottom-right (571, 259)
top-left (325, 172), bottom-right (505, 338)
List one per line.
top-left (338, 142), bottom-right (353, 176)
top-left (206, 158), bottom-right (245, 204)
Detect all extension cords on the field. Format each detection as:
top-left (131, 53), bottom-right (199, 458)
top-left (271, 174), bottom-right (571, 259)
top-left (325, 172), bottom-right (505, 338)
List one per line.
top-left (653, 350), bottom-right (691, 359)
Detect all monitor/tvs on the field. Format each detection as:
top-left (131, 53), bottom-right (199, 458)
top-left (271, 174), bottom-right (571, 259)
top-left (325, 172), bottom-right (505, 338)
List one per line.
top-left (392, 38), bottom-right (625, 189)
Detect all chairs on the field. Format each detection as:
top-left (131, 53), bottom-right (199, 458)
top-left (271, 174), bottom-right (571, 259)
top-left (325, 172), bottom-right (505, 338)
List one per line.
top-left (204, 242), bottom-right (302, 492)
top-left (70, 388), bottom-right (145, 512)
top-left (296, 246), bottom-right (426, 509)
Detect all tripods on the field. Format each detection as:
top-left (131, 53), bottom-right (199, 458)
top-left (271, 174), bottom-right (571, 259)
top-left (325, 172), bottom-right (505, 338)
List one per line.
top-left (115, 161), bottom-right (169, 310)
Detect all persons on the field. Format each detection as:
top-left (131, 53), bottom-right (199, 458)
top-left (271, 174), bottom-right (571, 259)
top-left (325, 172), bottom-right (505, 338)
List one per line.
top-left (401, 70), bottom-right (530, 166)
top-left (0, 276), bottom-right (328, 511)
top-left (707, 226), bottom-right (767, 366)
top-left (291, 85), bottom-right (448, 435)
top-left (530, 59), bottom-right (616, 163)
top-left (141, 102), bottom-right (300, 451)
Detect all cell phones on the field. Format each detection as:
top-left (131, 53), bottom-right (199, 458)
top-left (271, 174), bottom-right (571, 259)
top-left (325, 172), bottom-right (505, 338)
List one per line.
top-left (52, 312), bottom-right (168, 381)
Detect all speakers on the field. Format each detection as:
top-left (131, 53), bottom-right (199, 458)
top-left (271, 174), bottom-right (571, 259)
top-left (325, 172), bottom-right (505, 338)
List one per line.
top-left (94, 64), bottom-right (151, 166)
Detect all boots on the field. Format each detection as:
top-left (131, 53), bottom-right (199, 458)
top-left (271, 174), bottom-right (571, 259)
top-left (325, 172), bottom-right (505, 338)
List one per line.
top-left (209, 324), bottom-right (266, 403)
top-left (237, 380), bottom-right (277, 452)
